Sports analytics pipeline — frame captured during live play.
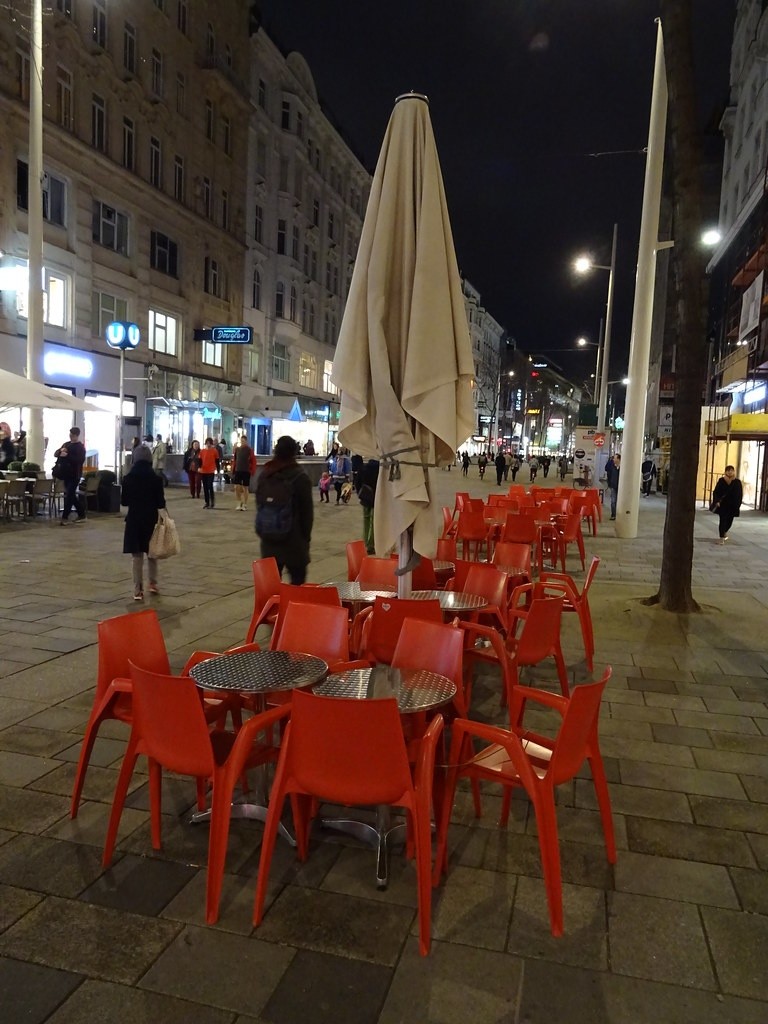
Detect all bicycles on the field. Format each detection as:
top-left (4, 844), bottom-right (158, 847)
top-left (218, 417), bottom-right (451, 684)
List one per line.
top-left (479, 462), bottom-right (485, 479)
top-left (531, 470), bottom-right (536, 482)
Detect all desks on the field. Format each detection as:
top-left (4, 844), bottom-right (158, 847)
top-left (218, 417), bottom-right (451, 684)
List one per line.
top-left (188, 650), bottom-right (329, 848)
top-left (432, 560), bottom-right (456, 572)
top-left (411, 590), bottom-right (490, 619)
top-left (316, 581), bottom-right (397, 617)
top-left (312, 666), bottom-right (457, 893)
top-left (496, 564), bottom-right (529, 577)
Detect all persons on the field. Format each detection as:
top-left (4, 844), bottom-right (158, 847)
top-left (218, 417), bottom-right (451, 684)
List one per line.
top-left (296, 440), bottom-right (315, 456)
top-left (254, 435), bottom-right (315, 587)
top-left (444, 447), bottom-right (576, 486)
top-left (197, 438), bottom-right (220, 509)
top-left (231, 436), bottom-right (257, 511)
top-left (54, 427), bottom-right (88, 527)
top-left (318, 443), bottom-right (364, 505)
top-left (642, 455), bottom-right (658, 500)
top-left (354, 458), bottom-right (380, 556)
top-left (709, 466), bottom-right (743, 545)
top-left (604, 453), bottom-right (621, 522)
top-left (121, 445), bottom-right (166, 600)
top-left (130, 432), bottom-right (169, 488)
top-left (183, 436), bottom-right (227, 500)
top-left (0, 422), bottom-right (27, 480)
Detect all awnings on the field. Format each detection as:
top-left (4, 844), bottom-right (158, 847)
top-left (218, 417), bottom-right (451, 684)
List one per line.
top-left (716, 377), bottom-right (767, 394)
top-left (748, 361), bottom-right (768, 373)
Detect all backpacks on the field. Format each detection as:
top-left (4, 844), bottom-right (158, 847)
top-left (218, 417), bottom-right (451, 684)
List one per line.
top-left (254, 470), bottom-right (302, 543)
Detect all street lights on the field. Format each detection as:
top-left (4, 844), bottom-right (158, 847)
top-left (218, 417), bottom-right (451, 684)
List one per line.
top-left (578, 338), bottom-right (601, 404)
top-left (494, 371), bottom-right (514, 461)
top-left (106, 320), bottom-right (140, 486)
top-left (576, 257), bottom-right (615, 488)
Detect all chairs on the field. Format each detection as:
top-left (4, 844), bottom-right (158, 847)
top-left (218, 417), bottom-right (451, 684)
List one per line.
top-left (0, 474), bottom-right (101, 525)
top-left (67, 484), bottom-right (616, 957)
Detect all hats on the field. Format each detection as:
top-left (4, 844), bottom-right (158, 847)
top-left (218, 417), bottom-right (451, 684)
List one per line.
top-left (270, 435), bottom-right (301, 458)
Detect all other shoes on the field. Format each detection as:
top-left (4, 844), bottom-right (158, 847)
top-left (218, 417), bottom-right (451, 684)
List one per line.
top-left (240, 504), bottom-right (248, 511)
top-left (148, 584), bottom-right (160, 596)
top-left (644, 495), bottom-right (649, 499)
top-left (73, 515), bottom-right (87, 523)
top-left (192, 493), bottom-right (200, 499)
top-left (203, 503), bottom-right (214, 509)
top-left (234, 505), bottom-right (242, 511)
top-left (609, 517), bottom-right (616, 522)
top-left (641, 492), bottom-right (645, 496)
top-left (723, 534), bottom-right (728, 540)
top-left (133, 591), bottom-right (143, 600)
top-left (719, 537), bottom-right (724, 545)
top-left (60, 518), bottom-right (73, 526)
top-left (319, 498), bottom-right (324, 502)
top-left (325, 500), bottom-right (329, 503)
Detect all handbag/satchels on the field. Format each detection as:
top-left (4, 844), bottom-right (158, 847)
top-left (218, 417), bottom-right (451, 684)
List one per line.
top-left (147, 509), bottom-right (182, 560)
top-left (709, 502), bottom-right (720, 515)
top-left (340, 479), bottom-right (352, 504)
top-left (642, 472), bottom-right (652, 482)
top-left (51, 458), bottom-right (65, 480)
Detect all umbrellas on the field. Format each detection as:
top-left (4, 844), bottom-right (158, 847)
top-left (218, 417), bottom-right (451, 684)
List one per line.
top-left (0, 368), bottom-right (110, 414)
top-left (331, 90), bottom-right (481, 602)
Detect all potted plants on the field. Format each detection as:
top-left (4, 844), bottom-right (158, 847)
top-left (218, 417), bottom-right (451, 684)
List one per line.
top-left (79, 470), bottom-right (121, 513)
top-left (0, 461), bottom-right (46, 481)
